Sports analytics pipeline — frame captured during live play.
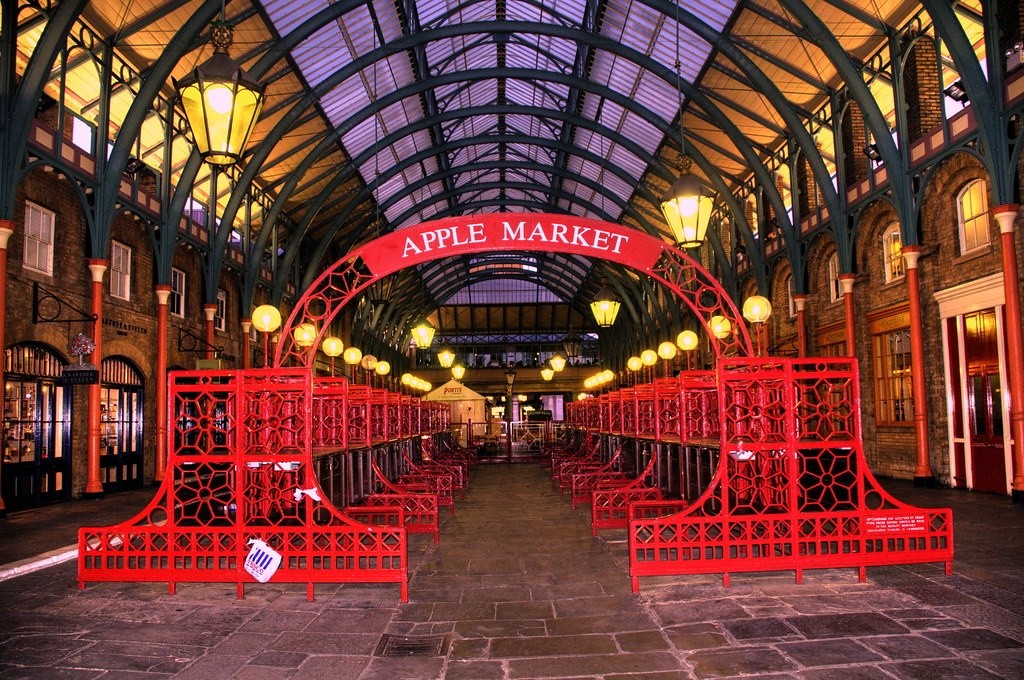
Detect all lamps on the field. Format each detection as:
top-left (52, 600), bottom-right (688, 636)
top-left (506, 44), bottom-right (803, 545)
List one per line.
top-left (171, 0), bottom-right (771, 400)
